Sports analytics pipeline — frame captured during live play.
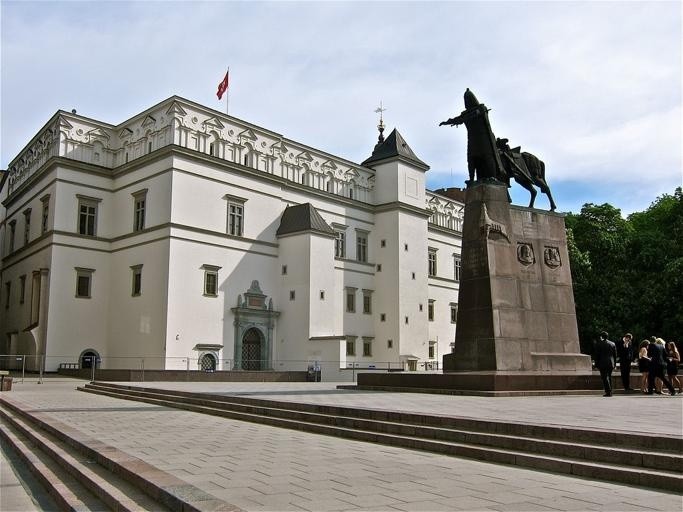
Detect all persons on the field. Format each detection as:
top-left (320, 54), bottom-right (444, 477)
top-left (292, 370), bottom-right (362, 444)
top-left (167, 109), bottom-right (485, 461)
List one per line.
top-left (654, 337), bottom-right (666, 395)
top-left (666, 341), bottom-right (683, 394)
top-left (645, 335), bottom-right (677, 396)
top-left (616, 332), bottom-right (635, 392)
top-left (639, 339), bottom-right (652, 394)
top-left (593, 331), bottom-right (617, 397)
top-left (313, 361), bottom-right (321, 371)
top-left (438, 87), bottom-right (509, 185)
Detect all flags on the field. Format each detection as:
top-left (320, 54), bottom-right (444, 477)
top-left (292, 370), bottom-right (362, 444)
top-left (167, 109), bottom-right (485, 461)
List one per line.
top-left (215, 69), bottom-right (228, 101)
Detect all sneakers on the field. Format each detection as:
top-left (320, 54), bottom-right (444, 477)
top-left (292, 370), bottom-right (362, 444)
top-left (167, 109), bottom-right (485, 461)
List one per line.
top-left (603, 387), bottom-right (682, 397)
